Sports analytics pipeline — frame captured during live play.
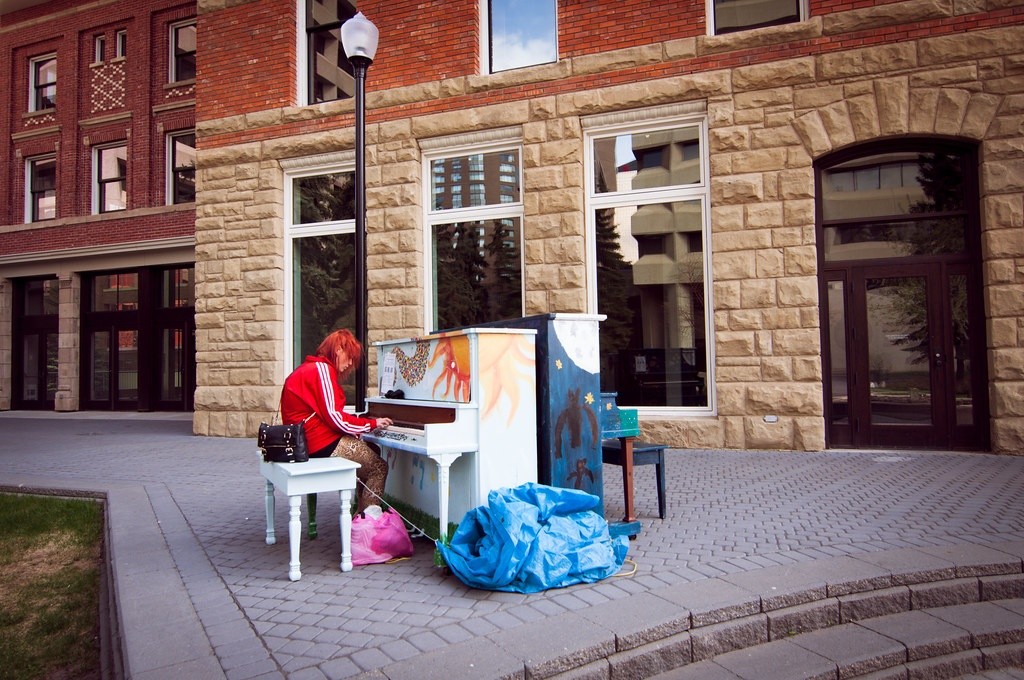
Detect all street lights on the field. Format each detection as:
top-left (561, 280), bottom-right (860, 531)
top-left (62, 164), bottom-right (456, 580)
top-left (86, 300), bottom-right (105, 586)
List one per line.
top-left (341, 10), bottom-right (379, 413)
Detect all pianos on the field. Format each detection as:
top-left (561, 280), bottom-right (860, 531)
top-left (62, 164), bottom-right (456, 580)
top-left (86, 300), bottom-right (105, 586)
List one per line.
top-left (338, 328), bottom-right (541, 571)
top-left (429, 311), bottom-right (640, 539)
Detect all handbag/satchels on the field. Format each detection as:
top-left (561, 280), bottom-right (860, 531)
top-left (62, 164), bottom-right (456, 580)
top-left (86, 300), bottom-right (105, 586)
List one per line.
top-left (351, 505), bottom-right (414, 565)
top-left (257, 422), bottom-right (309, 462)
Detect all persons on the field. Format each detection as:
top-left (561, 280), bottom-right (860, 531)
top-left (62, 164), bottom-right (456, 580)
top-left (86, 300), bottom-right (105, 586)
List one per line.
top-left (281, 329), bottom-right (394, 519)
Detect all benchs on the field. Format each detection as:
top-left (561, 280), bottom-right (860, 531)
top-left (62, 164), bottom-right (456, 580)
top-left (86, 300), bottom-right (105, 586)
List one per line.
top-left (255, 450), bottom-right (361, 581)
top-left (601, 439), bottom-right (668, 519)
